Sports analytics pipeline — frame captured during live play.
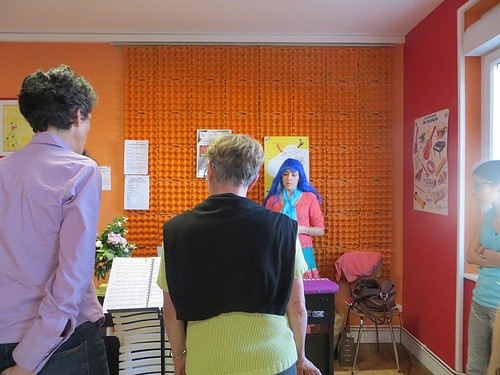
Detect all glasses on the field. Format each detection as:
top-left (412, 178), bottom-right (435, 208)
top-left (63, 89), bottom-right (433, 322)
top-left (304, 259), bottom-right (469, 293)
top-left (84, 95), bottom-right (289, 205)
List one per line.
top-left (204, 168), bottom-right (207, 181)
top-left (472, 181), bottom-right (494, 192)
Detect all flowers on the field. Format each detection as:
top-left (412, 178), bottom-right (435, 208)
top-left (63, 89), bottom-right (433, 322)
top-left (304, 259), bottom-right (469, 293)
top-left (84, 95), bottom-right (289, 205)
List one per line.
top-left (94, 216), bottom-right (138, 279)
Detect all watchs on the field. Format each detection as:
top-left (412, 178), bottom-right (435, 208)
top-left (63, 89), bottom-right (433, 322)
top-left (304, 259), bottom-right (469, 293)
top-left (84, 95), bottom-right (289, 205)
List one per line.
top-left (479, 248), bottom-right (485, 258)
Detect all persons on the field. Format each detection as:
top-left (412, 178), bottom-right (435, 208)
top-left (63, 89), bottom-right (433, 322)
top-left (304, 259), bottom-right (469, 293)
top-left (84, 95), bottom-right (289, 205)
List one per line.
top-left (466, 159), bottom-right (500, 375)
top-left (0, 64), bottom-right (111, 375)
top-left (156, 134), bottom-right (321, 375)
top-left (264, 158), bottom-right (326, 279)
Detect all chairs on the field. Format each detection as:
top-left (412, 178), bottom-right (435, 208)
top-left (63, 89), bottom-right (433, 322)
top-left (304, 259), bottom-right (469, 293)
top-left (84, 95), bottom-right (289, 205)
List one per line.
top-left (334, 252), bottom-right (401, 375)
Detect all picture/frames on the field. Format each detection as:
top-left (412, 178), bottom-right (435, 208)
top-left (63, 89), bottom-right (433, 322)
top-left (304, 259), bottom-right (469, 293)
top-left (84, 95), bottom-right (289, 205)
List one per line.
top-left (0, 98), bottom-right (36, 158)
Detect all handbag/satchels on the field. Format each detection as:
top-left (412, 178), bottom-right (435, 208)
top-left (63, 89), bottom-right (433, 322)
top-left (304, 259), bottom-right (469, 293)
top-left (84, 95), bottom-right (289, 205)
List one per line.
top-left (353, 279), bottom-right (396, 325)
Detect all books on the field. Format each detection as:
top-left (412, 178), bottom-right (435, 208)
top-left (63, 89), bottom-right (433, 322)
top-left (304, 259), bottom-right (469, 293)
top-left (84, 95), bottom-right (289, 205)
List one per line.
top-left (103, 256), bottom-right (177, 375)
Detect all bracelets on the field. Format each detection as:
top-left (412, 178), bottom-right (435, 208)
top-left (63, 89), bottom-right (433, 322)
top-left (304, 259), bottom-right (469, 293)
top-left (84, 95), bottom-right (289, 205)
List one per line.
top-left (171, 350), bottom-right (186, 359)
